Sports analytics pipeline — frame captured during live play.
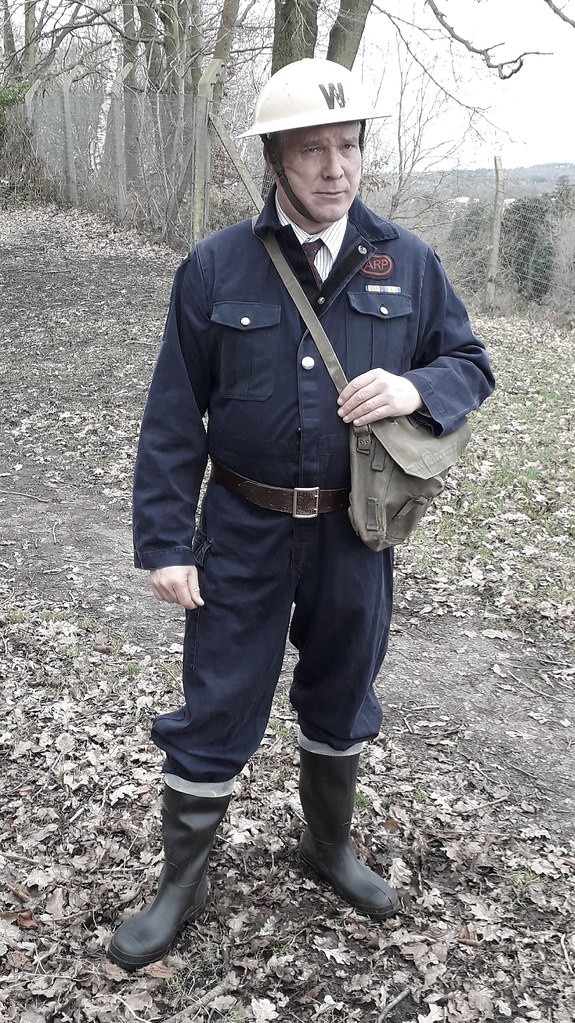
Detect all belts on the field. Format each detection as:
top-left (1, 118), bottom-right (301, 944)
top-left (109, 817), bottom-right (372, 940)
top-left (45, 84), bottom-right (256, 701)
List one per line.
top-left (209, 457), bottom-right (349, 519)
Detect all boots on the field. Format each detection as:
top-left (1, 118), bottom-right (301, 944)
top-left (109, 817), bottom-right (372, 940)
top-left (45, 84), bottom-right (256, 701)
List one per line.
top-left (110, 774), bottom-right (237, 972)
top-left (295, 726), bottom-right (399, 915)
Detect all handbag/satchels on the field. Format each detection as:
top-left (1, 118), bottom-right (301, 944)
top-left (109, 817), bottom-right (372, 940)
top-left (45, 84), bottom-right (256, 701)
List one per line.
top-left (348, 414), bottom-right (472, 552)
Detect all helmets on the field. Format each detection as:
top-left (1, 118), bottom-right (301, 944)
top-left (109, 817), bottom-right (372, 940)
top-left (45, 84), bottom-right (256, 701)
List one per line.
top-left (235, 56), bottom-right (392, 138)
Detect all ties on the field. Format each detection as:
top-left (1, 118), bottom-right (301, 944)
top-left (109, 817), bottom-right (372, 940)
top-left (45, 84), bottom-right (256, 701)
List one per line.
top-left (300, 237), bottom-right (324, 292)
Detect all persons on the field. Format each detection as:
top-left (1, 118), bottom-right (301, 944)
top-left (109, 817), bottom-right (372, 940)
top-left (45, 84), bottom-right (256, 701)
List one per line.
top-left (109, 57), bottom-right (496, 970)
